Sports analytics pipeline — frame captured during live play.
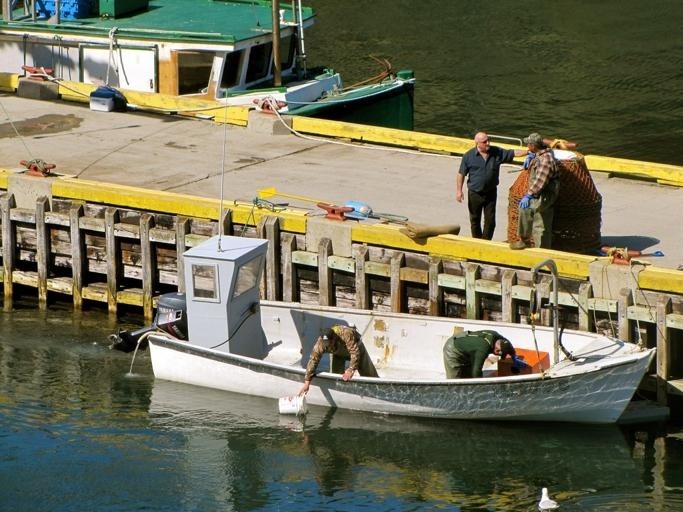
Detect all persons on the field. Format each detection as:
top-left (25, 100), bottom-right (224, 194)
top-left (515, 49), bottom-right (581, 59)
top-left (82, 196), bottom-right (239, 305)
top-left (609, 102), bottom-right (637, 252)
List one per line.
top-left (457, 132), bottom-right (533, 241)
top-left (298, 323), bottom-right (380, 398)
top-left (510, 133), bottom-right (560, 249)
top-left (443, 330), bottom-right (529, 379)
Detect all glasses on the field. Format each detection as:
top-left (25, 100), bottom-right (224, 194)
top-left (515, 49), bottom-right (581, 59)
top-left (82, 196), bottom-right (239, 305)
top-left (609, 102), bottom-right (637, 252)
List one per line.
top-left (480, 139), bottom-right (490, 144)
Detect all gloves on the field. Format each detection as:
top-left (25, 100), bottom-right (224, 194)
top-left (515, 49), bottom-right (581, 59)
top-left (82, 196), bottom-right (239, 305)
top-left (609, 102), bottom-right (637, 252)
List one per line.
top-left (520, 194), bottom-right (532, 208)
top-left (511, 354), bottom-right (527, 368)
top-left (524, 153), bottom-right (535, 171)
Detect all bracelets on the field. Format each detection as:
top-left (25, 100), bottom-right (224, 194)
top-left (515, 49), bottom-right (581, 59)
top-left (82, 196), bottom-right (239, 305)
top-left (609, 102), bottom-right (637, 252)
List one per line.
top-left (527, 148), bottom-right (530, 155)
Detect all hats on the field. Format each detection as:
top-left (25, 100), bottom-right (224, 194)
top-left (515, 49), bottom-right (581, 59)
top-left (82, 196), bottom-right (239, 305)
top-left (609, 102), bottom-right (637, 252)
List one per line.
top-left (523, 133), bottom-right (543, 144)
top-left (322, 328), bottom-right (333, 346)
top-left (501, 338), bottom-right (509, 359)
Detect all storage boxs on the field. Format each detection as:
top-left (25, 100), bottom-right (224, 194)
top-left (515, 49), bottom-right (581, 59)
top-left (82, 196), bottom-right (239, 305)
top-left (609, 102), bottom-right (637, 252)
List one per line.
top-left (89, 97), bottom-right (114, 112)
top-left (497, 348), bottom-right (551, 376)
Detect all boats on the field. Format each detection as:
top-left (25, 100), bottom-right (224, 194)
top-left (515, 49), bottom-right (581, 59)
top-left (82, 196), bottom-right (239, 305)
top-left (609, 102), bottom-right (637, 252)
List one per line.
top-left (0, 0), bottom-right (416, 138)
top-left (108, 89), bottom-right (658, 429)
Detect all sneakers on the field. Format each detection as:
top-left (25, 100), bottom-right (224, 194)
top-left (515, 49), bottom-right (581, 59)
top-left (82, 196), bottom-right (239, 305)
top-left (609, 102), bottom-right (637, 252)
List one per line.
top-left (511, 240), bottom-right (529, 249)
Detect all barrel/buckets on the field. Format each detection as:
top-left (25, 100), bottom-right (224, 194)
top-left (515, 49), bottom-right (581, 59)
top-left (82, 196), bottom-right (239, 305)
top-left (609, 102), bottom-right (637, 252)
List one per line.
top-left (278, 393), bottom-right (308, 417)
top-left (278, 413), bottom-right (305, 432)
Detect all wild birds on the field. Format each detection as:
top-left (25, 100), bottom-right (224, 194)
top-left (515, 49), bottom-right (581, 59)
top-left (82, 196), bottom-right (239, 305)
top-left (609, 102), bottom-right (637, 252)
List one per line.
top-left (538, 486), bottom-right (559, 512)
